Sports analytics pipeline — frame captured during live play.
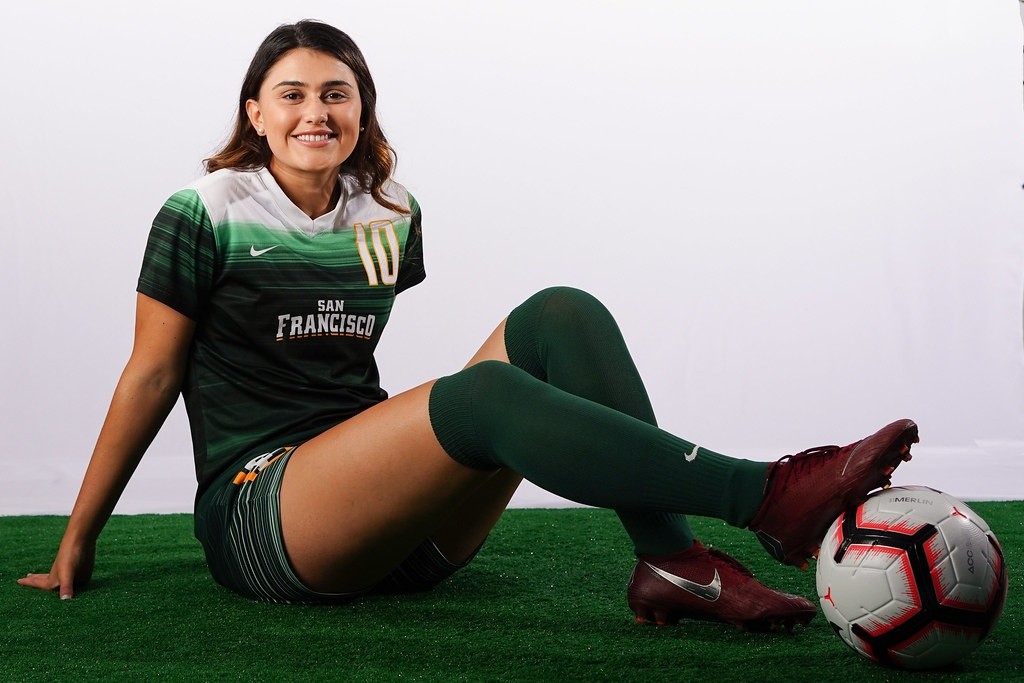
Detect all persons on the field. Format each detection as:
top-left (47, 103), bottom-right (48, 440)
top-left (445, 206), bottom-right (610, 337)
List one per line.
top-left (14, 16), bottom-right (921, 635)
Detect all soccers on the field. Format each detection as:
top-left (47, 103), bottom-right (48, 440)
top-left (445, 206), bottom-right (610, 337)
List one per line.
top-left (815, 485), bottom-right (1008, 669)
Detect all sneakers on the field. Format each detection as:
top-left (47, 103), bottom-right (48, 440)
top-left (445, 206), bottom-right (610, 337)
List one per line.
top-left (746, 418), bottom-right (920, 571)
top-left (626, 539), bottom-right (817, 634)
